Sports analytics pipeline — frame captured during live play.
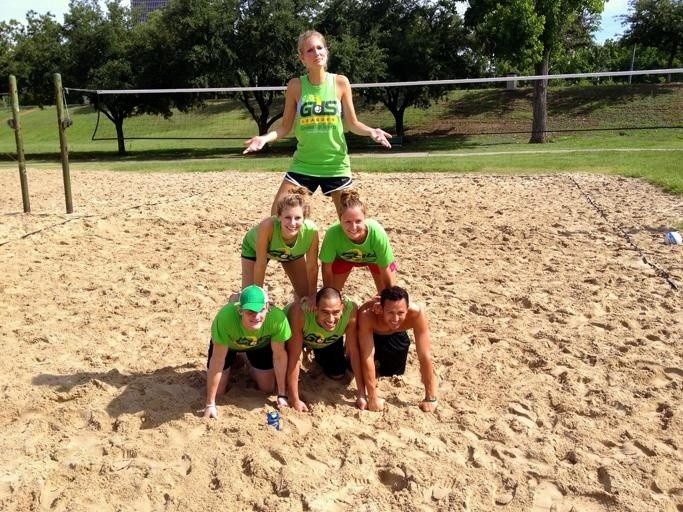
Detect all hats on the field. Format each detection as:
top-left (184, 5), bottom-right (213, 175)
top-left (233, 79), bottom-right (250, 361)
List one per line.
top-left (238, 285), bottom-right (267, 313)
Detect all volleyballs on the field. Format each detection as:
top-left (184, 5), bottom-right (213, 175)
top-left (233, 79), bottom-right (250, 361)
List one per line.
top-left (664, 232), bottom-right (683, 245)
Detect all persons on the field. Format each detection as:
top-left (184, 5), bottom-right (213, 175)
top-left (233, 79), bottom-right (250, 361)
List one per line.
top-left (242, 30), bottom-right (393, 222)
top-left (205, 284), bottom-right (295, 419)
top-left (240, 186), bottom-right (318, 314)
top-left (282, 287), bottom-right (370, 412)
top-left (357, 286), bottom-right (437, 413)
top-left (318, 187), bottom-right (396, 316)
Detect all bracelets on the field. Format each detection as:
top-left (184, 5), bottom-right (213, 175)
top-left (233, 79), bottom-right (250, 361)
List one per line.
top-left (206, 404), bottom-right (214, 408)
top-left (278, 395), bottom-right (288, 400)
top-left (425, 398), bottom-right (436, 402)
